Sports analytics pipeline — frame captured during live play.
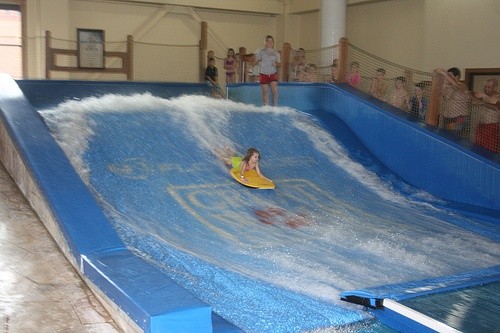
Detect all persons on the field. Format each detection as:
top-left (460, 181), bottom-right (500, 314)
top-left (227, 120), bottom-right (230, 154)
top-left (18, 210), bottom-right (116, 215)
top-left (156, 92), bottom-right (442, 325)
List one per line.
top-left (365, 68), bottom-right (387, 101)
top-left (405, 82), bottom-right (427, 121)
top-left (235, 47), bottom-right (257, 82)
top-left (249, 35), bottom-right (280, 107)
top-left (205, 58), bottom-right (226, 98)
top-left (210, 146), bottom-right (272, 183)
top-left (287, 48), bottom-right (318, 82)
top-left (434, 67), bottom-right (471, 135)
top-left (388, 76), bottom-right (408, 109)
top-left (471, 78), bottom-right (500, 153)
top-left (224, 49), bottom-right (236, 83)
top-left (345, 62), bottom-right (361, 88)
top-left (330, 59), bottom-right (337, 80)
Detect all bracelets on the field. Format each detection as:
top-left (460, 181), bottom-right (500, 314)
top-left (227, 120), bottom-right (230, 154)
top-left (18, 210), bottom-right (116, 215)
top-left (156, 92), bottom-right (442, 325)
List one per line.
top-left (240, 176), bottom-right (244, 178)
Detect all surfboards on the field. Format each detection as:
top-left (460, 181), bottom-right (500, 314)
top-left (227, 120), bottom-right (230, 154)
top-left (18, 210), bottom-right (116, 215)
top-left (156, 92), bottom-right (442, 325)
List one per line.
top-left (231, 168), bottom-right (276, 189)
